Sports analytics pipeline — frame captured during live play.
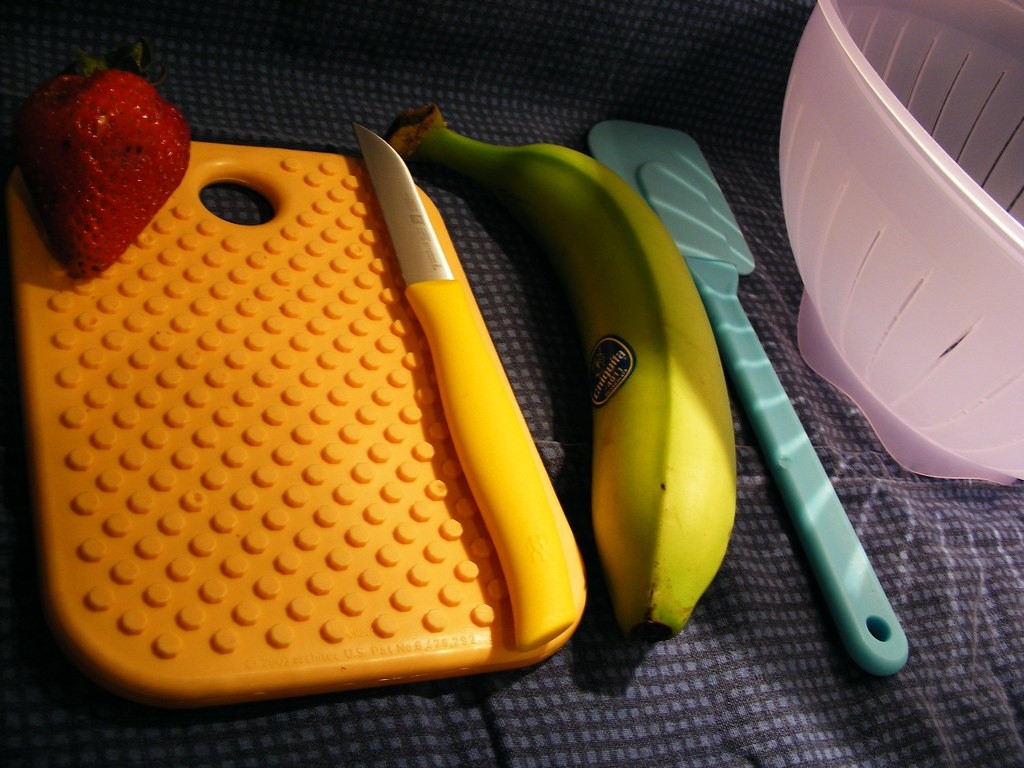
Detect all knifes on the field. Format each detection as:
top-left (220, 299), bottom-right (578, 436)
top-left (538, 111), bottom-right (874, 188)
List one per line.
top-left (352, 122), bottom-right (588, 652)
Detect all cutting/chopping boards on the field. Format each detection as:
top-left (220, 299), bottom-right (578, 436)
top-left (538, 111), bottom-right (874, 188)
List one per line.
top-left (9, 141), bottom-right (585, 706)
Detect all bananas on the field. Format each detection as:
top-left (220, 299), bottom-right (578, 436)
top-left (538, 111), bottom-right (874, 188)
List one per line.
top-left (383, 105), bottom-right (736, 639)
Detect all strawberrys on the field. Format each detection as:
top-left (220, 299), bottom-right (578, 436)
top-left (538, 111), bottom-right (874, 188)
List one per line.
top-left (21, 69), bottom-right (190, 277)
